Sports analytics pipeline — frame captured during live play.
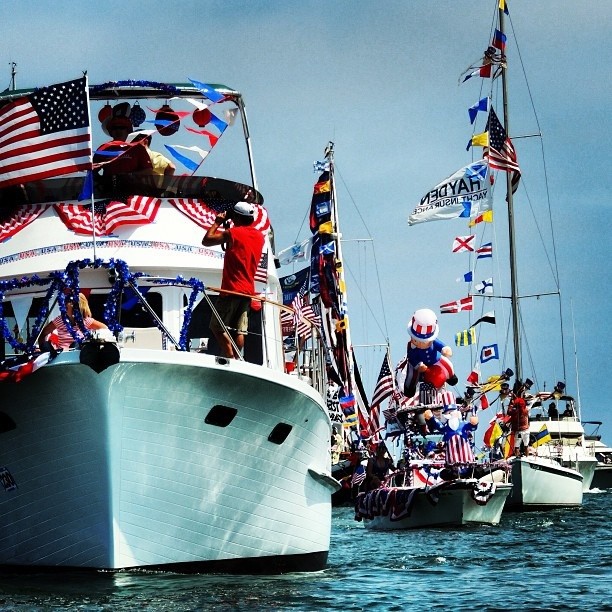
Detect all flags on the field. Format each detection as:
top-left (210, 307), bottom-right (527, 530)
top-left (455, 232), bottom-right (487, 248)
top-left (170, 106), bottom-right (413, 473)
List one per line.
top-left (466, 132), bottom-right (490, 151)
top-left (452, 234), bottom-right (474, 254)
top-left (319, 222), bottom-right (333, 235)
top-left (407, 157), bottom-right (494, 226)
top-left (340, 392), bottom-right (361, 429)
top-left (368, 353), bottom-right (396, 411)
top-left (467, 309), bottom-right (495, 330)
top-left (475, 277), bottom-right (493, 295)
top-left (318, 241), bottom-right (335, 255)
top-left (467, 95), bottom-right (489, 125)
top-left (459, 61), bottom-right (493, 84)
top-left (468, 370), bottom-right (479, 386)
top-left (203, 200), bottom-right (266, 363)
top-left (469, 211), bottom-right (494, 227)
top-left (480, 105), bottom-right (524, 204)
top-left (278, 236), bottom-right (314, 265)
top-left (534, 425), bottom-right (549, 449)
top-left (455, 327), bottom-right (477, 347)
top-left (280, 273), bottom-right (323, 340)
top-left (314, 180), bottom-right (330, 193)
top-left (315, 202), bottom-right (330, 217)
top-left (473, 243), bottom-right (494, 259)
top-left (313, 160), bottom-right (330, 171)
top-left (480, 344), bottom-right (500, 362)
top-left (480, 393), bottom-right (489, 410)
top-left (457, 271), bottom-right (474, 283)
top-left (0, 77), bottom-right (93, 187)
top-left (440, 296), bottom-right (473, 314)
top-left (367, 405), bottom-right (381, 440)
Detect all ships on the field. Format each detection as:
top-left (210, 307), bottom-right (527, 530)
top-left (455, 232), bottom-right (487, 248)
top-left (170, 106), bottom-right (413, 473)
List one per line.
top-left (581, 420), bottom-right (612, 490)
top-left (410, 2), bottom-right (598, 508)
top-left (359, 385), bottom-right (513, 529)
top-left (278, 142), bottom-right (379, 507)
top-left (1, 61), bottom-right (343, 574)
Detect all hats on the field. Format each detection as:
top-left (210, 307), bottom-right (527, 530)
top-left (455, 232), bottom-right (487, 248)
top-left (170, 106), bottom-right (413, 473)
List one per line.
top-left (232, 201), bottom-right (255, 216)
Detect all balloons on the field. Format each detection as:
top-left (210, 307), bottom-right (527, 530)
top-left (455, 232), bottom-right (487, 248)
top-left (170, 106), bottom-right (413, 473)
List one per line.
top-left (401, 308), bottom-right (458, 399)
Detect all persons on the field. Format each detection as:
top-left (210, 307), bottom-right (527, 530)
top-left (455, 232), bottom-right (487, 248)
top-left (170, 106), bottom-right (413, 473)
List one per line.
top-left (40, 294), bottom-right (114, 358)
